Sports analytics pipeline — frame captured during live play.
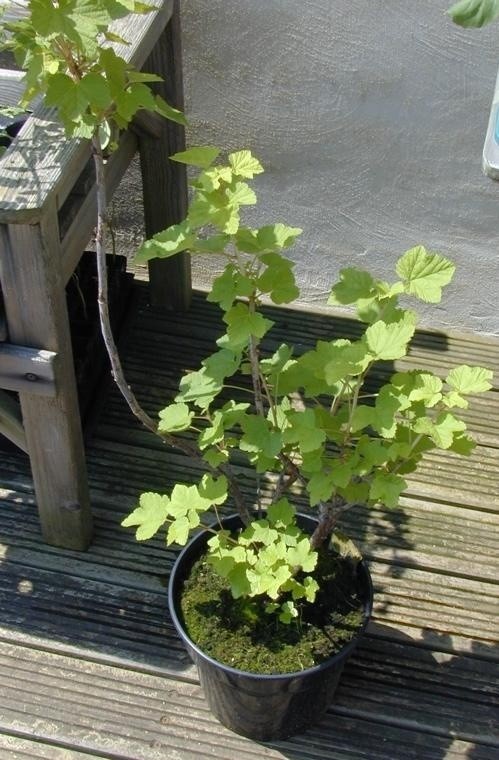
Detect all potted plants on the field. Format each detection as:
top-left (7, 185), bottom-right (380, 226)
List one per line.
top-left (0, 0), bottom-right (494, 742)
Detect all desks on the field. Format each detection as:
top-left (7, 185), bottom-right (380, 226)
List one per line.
top-left (0, 0), bottom-right (192, 553)
